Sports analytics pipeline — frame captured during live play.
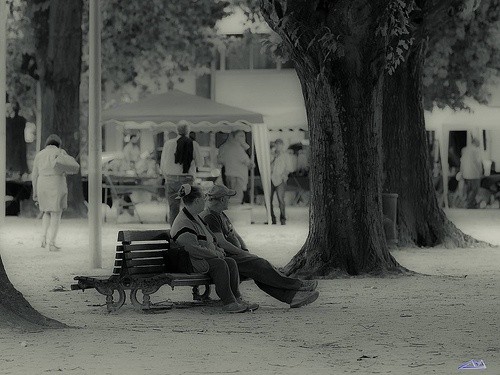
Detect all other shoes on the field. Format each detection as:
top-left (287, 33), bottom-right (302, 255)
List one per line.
top-left (298, 279), bottom-right (318, 291)
top-left (223, 302), bottom-right (248, 313)
top-left (280, 217), bottom-right (285, 225)
top-left (265, 216), bottom-right (276, 224)
top-left (290, 290), bottom-right (319, 308)
top-left (237, 296), bottom-right (259, 312)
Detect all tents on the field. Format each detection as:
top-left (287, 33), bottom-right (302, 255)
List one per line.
top-left (93, 87), bottom-right (267, 227)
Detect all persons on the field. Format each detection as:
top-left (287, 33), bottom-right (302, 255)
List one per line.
top-left (31, 133), bottom-right (79, 252)
top-left (169, 184), bottom-right (261, 314)
top-left (431, 130), bottom-right (500, 209)
top-left (94, 124), bottom-right (307, 227)
top-left (198, 185), bottom-right (319, 308)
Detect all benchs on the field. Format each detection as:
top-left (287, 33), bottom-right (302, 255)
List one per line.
top-left (71, 230), bottom-right (214, 314)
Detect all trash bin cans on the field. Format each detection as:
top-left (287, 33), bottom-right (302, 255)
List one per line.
top-left (382, 192), bottom-right (399, 242)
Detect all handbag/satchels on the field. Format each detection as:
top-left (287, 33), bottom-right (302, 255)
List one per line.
top-left (53, 149), bottom-right (80, 175)
top-left (162, 247), bottom-right (193, 275)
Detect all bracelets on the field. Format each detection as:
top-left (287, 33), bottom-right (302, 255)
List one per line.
top-left (241, 248), bottom-right (249, 252)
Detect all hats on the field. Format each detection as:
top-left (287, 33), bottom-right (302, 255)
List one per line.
top-left (45, 134), bottom-right (62, 146)
top-left (206, 184), bottom-right (238, 196)
